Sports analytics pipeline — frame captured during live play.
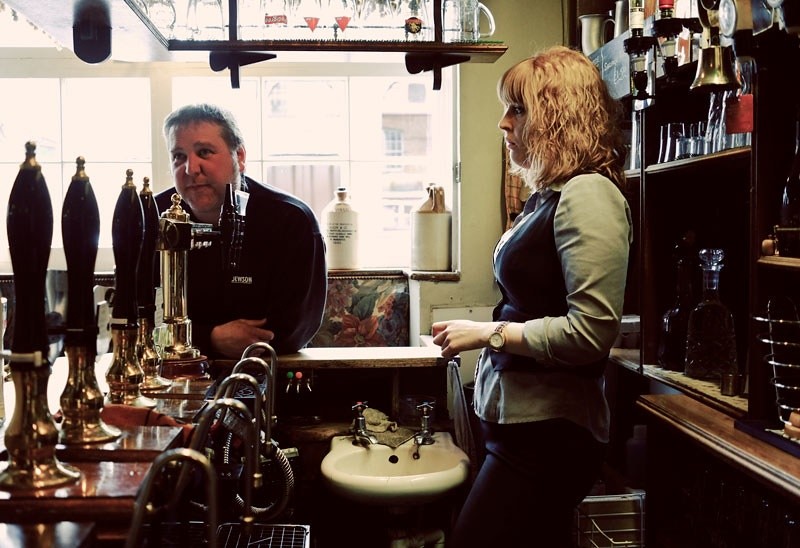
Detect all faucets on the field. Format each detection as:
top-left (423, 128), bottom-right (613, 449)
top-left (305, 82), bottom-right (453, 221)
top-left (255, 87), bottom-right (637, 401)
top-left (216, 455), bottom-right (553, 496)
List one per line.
top-left (352, 401), bottom-right (378, 447)
top-left (413, 400), bottom-right (437, 446)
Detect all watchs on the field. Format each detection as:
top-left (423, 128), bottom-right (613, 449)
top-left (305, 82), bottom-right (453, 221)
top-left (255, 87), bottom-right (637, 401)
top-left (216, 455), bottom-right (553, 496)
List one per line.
top-left (489, 321), bottom-right (506, 353)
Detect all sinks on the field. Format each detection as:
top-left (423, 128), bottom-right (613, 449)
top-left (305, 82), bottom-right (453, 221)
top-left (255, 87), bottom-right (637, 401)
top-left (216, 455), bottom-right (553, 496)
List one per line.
top-left (321, 443), bottom-right (471, 500)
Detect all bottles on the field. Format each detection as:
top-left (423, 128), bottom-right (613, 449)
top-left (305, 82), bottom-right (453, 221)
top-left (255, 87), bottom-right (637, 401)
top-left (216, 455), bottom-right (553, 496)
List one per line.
top-left (654, 230), bottom-right (739, 378)
top-left (321, 188), bottom-right (357, 271)
top-left (412, 183), bottom-right (451, 271)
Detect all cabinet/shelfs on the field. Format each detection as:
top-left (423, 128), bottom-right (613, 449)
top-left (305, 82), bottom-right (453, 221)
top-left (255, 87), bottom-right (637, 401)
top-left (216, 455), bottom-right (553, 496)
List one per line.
top-left (584, 0), bottom-right (800, 548)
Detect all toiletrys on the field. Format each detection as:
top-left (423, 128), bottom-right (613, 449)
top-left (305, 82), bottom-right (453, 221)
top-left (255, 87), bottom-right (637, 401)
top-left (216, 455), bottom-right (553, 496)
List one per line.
top-left (409, 182), bottom-right (452, 273)
top-left (320, 187), bottom-right (360, 271)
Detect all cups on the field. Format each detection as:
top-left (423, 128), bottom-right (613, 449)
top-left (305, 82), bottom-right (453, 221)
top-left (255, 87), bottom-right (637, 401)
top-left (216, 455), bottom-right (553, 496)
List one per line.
top-left (721, 372), bottom-right (742, 396)
top-left (658, 87), bottom-right (743, 163)
top-left (136, 0), bottom-right (497, 44)
top-left (578, 13), bottom-right (616, 56)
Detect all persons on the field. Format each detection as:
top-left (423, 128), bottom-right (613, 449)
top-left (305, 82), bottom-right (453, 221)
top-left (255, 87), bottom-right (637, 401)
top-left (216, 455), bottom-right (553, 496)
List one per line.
top-left (156, 109), bottom-right (327, 358)
top-left (430, 49), bottom-right (633, 548)
